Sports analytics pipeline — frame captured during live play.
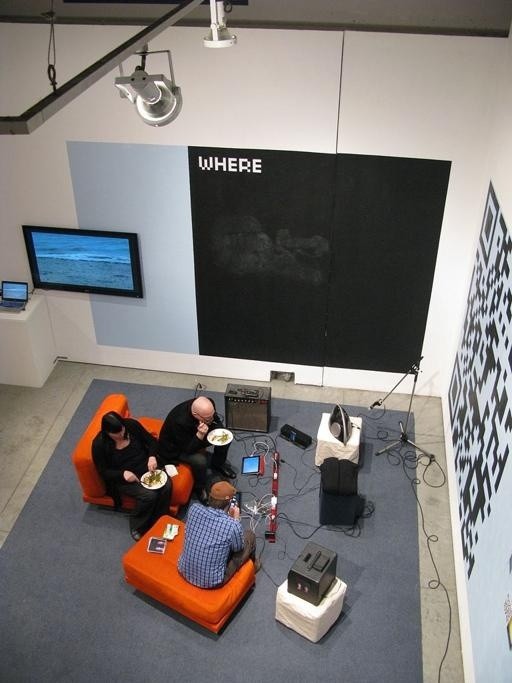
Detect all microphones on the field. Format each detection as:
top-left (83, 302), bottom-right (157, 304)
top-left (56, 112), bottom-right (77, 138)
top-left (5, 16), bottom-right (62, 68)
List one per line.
top-left (368, 401), bottom-right (380, 411)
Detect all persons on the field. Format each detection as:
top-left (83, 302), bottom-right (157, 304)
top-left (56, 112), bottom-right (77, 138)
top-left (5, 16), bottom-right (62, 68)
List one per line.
top-left (159, 395), bottom-right (236, 498)
top-left (92, 411), bottom-right (172, 541)
top-left (176, 481), bottom-right (263, 589)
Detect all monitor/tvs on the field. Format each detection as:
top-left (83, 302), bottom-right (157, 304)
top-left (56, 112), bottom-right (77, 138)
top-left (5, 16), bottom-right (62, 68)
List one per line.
top-left (21, 224), bottom-right (144, 299)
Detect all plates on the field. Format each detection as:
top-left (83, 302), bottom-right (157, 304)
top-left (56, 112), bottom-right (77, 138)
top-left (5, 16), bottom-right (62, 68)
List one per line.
top-left (140, 469), bottom-right (169, 490)
top-left (206, 428), bottom-right (233, 447)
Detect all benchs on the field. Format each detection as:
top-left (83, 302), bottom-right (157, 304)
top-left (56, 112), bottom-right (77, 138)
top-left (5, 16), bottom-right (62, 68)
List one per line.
top-left (123, 515), bottom-right (255, 637)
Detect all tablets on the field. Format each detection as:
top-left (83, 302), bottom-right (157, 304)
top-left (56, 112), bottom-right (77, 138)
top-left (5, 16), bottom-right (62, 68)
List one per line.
top-left (241, 456), bottom-right (259, 474)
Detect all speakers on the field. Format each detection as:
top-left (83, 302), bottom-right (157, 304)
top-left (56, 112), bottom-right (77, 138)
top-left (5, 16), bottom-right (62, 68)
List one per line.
top-left (287, 540), bottom-right (338, 606)
top-left (224, 383), bottom-right (271, 433)
top-left (328, 403), bottom-right (353, 445)
top-left (318, 456), bottom-right (358, 530)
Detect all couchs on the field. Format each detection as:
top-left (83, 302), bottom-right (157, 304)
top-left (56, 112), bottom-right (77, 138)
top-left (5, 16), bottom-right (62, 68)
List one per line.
top-left (72, 394), bottom-right (193, 515)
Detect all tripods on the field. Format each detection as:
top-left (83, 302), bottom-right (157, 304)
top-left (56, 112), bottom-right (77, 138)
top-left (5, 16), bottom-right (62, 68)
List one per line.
top-left (374, 355), bottom-right (435, 459)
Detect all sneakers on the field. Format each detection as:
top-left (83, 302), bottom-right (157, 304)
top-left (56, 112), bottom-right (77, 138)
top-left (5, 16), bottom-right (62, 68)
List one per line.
top-left (129, 523), bottom-right (140, 541)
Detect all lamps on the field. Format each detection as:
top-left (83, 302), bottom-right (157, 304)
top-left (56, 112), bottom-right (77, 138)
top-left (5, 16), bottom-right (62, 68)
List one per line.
top-left (113, 42), bottom-right (184, 129)
top-left (201, 2), bottom-right (239, 48)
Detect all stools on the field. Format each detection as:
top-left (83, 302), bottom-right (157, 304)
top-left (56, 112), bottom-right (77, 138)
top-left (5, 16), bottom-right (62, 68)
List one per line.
top-left (273, 577), bottom-right (349, 645)
top-left (317, 413), bottom-right (364, 467)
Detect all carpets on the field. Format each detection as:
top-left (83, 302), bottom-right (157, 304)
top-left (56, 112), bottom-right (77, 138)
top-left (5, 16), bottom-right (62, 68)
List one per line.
top-left (0, 378), bottom-right (421, 683)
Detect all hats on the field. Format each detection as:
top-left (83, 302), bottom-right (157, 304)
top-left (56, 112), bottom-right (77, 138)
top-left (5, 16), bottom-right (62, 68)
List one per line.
top-left (208, 479), bottom-right (238, 502)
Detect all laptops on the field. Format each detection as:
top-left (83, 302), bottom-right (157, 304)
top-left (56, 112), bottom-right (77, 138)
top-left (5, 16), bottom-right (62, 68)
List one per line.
top-left (0, 280), bottom-right (28, 313)
